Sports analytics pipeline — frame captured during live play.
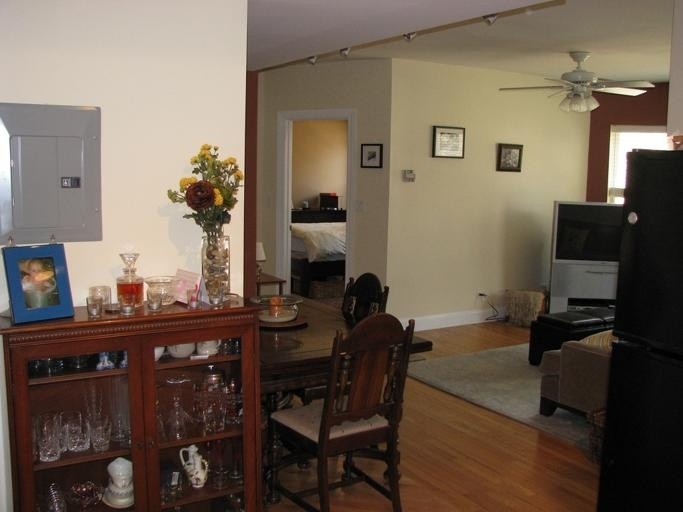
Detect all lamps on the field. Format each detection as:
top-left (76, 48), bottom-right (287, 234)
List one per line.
top-left (307, 13), bottom-right (499, 63)
top-left (256, 240), bottom-right (267, 280)
top-left (559, 92), bottom-right (601, 112)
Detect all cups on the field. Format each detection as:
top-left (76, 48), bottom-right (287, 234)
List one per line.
top-left (86, 295), bottom-right (102, 319)
top-left (119, 293), bottom-right (135, 316)
top-left (146, 288), bottom-right (162, 309)
top-left (209, 288), bottom-right (224, 310)
top-left (143, 276), bottom-right (177, 306)
top-left (185, 289), bottom-right (202, 312)
top-left (90, 286), bottom-right (111, 307)
top-left (31, 338), bottom-right (243, 510)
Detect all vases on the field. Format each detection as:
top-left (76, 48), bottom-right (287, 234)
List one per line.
top-left (200, 237), bottom-right (231, 307)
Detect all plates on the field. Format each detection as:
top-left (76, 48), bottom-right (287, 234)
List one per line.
top-left (105, 309), bottom-right (119, 313)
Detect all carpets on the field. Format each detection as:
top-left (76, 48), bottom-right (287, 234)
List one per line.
top-left (407, 342), bottom-right (594, 448)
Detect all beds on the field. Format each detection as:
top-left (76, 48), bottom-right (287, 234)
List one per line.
top-left (291, 221), bottom-right (346, 296)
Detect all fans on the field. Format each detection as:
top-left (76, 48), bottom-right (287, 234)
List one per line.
top-left (498, 49), bottom-right (656, 99)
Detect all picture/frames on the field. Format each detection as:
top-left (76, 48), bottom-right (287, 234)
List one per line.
top-left (496, 144), bottom-right (523, 172)
top-left (433, 126), bottom-right (466, 158)
top-left (361, 143), bottom-right (383, 168)
top-left (1, 244), bottom-right (74, 326)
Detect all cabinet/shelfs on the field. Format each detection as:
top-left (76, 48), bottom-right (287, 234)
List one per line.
top-left (0, 299), bottom-right (263, 512)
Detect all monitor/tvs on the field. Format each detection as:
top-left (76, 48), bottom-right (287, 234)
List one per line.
top-left (551, 200), bottom-right (623, 266)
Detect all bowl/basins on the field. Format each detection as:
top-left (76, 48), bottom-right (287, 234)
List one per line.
top-left (248, 295), bottom-right (304, 323)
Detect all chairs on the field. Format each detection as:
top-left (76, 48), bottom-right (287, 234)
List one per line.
top-left (286, 270), bottom-right (390, 404)
top-left (269, 309), bottom-right (415, 511)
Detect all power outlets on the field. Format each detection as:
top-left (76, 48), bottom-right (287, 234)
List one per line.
top-left (477, 289), bottom-right (486, 299)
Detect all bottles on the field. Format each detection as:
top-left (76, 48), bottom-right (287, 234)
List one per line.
top-left (116, 252), bottom-right (143, 307)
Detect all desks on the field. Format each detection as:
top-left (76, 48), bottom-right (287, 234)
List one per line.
top-left (257, 292), bottom-right (433, 448)
top-left (256, 271), bottom-right (287, 296)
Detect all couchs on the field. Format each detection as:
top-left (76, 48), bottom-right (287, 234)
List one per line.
top-left (537, 329), bottom-right (614, 417)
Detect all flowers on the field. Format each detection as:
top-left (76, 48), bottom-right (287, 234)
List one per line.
top-left (168, 143), bottom-right (244, 297)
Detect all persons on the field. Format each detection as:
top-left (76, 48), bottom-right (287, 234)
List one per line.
top-left (22, 260), bottom-right (53, 293)
